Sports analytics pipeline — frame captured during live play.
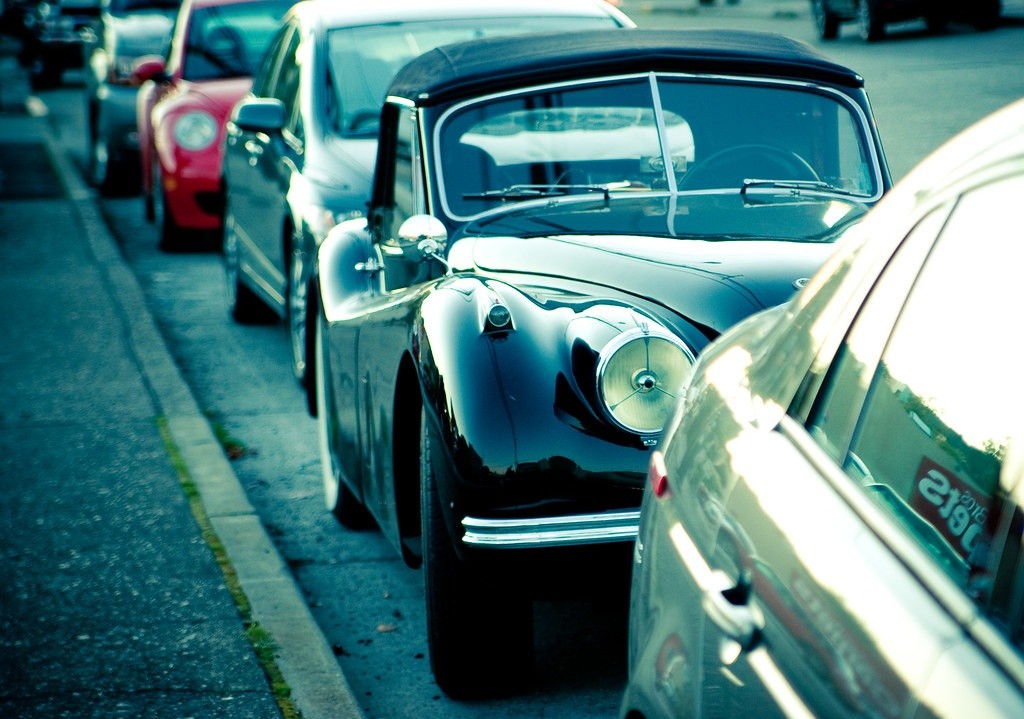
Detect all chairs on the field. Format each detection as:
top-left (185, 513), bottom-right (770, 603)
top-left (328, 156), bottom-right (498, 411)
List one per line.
top-left (702, 121), bottom-right (812, 188)
top-left (441, 143), bottom-right (503, 216)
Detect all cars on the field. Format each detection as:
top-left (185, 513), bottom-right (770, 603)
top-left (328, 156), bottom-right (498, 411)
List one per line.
top-left (311, 27), bottom-right (900, 703)
top-left (23, 0), bottom-right (1024, 719)
